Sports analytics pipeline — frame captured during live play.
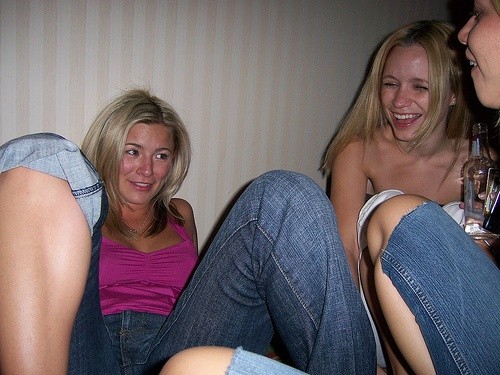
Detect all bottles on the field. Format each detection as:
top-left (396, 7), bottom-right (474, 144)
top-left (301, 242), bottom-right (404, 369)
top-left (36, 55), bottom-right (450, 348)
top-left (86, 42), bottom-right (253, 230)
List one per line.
top-left (463, 122), bottom-right (499, 223)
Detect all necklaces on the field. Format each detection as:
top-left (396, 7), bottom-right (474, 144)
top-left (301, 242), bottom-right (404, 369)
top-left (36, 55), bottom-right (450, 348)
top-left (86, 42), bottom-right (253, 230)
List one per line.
top-left (133, 210), bottom-right (152, 231)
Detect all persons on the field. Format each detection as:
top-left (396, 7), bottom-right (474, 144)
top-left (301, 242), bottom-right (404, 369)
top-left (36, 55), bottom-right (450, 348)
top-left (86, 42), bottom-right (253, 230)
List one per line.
top-left (367, 0), bottom-right (500, 375)
top-left (321, 19), bottom-right (500, 375)
top-left (0, 89), bottom-right (377, 375)
top-left (160, 345), bottom-right (310, 375)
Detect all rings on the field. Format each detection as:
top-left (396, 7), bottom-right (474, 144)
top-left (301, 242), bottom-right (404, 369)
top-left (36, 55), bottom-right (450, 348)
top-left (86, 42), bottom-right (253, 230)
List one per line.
top-left (484, 239), bottom-right (490, 247)
